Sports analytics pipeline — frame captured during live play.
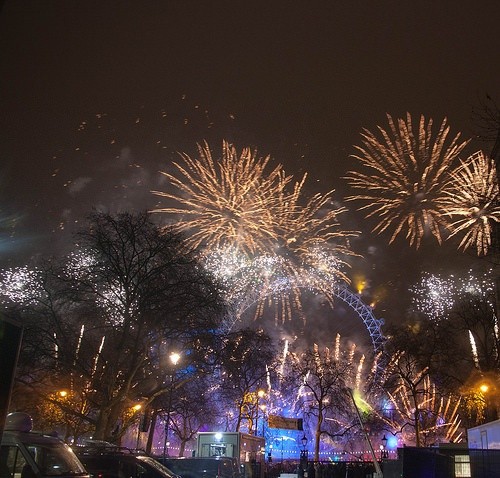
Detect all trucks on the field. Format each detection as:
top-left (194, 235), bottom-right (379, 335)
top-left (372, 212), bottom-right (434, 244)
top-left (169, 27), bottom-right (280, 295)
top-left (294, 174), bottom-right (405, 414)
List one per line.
top-left (195, 432), bottom-right (265, 478)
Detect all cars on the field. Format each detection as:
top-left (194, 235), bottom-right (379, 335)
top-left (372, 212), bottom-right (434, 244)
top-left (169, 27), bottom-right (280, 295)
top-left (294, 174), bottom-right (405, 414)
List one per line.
top-left (0, 412), bottom-right (240, 478)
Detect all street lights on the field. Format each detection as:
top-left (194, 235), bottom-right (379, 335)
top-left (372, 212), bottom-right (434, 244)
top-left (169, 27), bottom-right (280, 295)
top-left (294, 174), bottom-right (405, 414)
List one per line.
top-left (380, 433), bottom-right (388, 459)
top-left (301, 434), bottom-right (308, 460)
